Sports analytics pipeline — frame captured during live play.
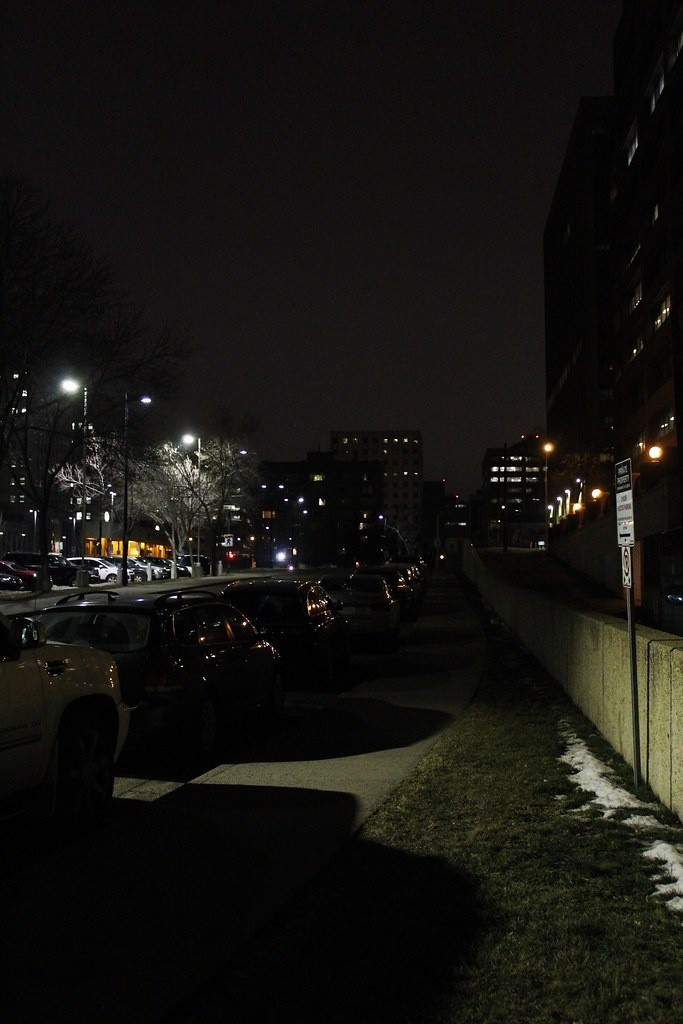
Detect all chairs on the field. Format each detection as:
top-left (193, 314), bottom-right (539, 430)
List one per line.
top-left (257, 599), bottom-right (303, 614)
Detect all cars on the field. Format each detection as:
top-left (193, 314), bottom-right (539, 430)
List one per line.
top-left (222, 555), bottom-right (429, 688)
top-left (0, 543), bottom-right (312, 591)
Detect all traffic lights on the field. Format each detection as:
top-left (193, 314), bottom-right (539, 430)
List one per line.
top-left (225, 538), bottom-right (233, 547)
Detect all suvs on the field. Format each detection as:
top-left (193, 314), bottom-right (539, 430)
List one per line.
top-left (36, 588), bottom-right (282, 761)
top-left (0, 610), bottom-right (130, 839)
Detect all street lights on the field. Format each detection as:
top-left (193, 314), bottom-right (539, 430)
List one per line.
top-left (261, 484), bottom-right (284, 569)
top-left (30, 509), bottom-right (40, 552)
top-left (61, 380), bottom-right (90, 589)
top-left (182, 434), bottom-right (204, 577)
top-left (542, 441), bottom-right (555, 551)
top-left (110, 492), bottom-right (117, 530)
top-left (121, 388), bottom-right (151, 585)
top-left (289, 537), bottom-right (293, 565)
